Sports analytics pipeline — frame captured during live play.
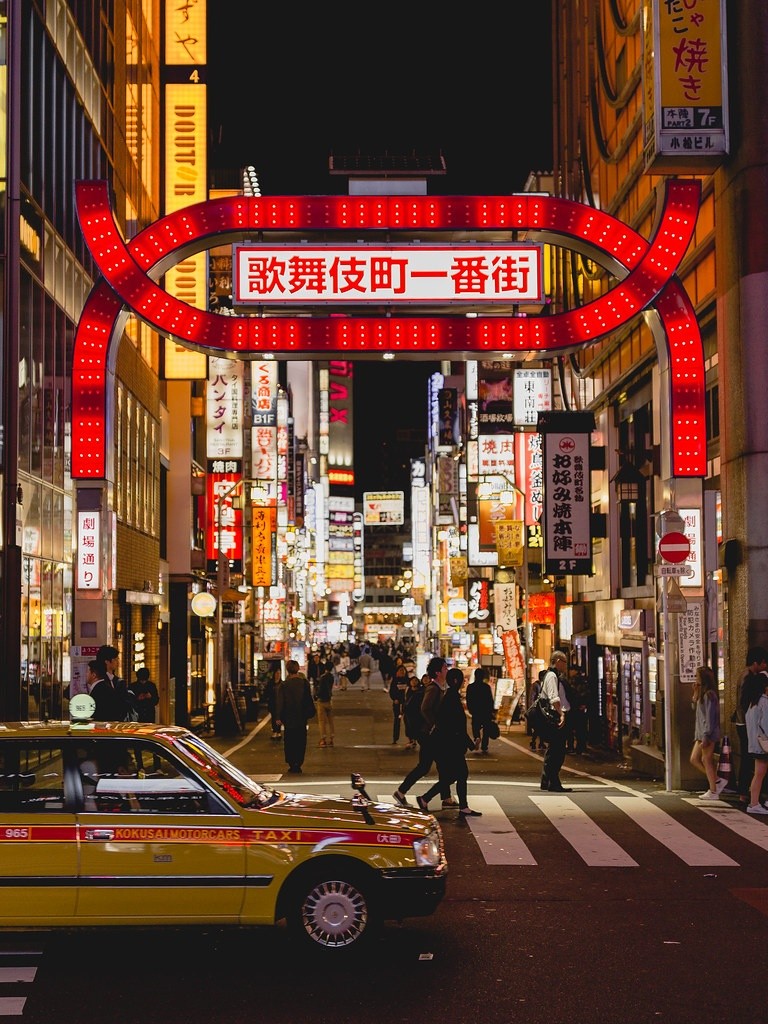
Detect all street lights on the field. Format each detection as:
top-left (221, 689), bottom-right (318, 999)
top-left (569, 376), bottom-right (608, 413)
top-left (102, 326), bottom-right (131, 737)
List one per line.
top-left (213, 478), bottom-right (275, 702)
top-left (470, 472), bottom-right (531, 735)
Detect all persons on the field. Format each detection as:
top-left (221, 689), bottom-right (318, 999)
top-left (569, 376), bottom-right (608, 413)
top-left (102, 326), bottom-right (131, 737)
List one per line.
top-left (732, 646), bottom-right (768, 815)
top-left (86, 645), bottom-right (162, 813)
top-left (267, 638), bottom-right (587, 817)
top-left (689, 666), bottom-right (729, 801)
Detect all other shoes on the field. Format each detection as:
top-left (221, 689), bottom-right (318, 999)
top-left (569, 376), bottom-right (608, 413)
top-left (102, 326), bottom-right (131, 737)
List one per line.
top-left (407, 742), bottom-right (416, 747)
top-left (288, 766), bottom-right (301, 773)
top-left (566, 748), bottom-right (574, 753)
top-left (482, 750), bottom-right (487, 753)
top-left (459, 809), bottom-right (482, 816)
top-left (575, 749), bottom-right (581, 755)
top-left (392, 740), bottom-right (397, 744)
top-left (315, 741), bottom-right (326, 748)
top-left (555, 788), bottom-right (572, 792)
top-left (270, 735), bottom-right (281, 740)
top-left (531, 745), bottom-right (536, 749)
top-left (416, 796), bottom-right (428, 812)
top-left (539, 745), bottom-right (546, 749)
top-left (326, 741), bottom-right (334, 747)
top-left (474, 738), bottom-right (480, 750)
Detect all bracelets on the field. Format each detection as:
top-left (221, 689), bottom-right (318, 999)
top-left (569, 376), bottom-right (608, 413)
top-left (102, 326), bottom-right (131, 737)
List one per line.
top-left (692, 696), bottom-right (697, 701)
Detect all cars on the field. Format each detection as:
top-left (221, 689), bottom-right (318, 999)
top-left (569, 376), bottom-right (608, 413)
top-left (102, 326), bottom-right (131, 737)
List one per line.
top-left (1, 692), bottom-right (447, 958)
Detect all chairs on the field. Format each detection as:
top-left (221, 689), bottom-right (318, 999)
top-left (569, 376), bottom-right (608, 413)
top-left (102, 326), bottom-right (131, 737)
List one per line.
top-left (77, 742), bottom-right (133, 811)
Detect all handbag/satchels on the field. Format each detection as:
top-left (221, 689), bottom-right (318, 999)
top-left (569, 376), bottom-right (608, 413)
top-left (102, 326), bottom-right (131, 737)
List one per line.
top-left (523, 698), bottom-right (559, 738)
top-left (487, 719), bottom-right (500, 739)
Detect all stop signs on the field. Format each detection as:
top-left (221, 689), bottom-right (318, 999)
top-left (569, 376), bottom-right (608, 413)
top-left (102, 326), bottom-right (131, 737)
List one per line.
top-left (659, 532), bottom-right (690, 563)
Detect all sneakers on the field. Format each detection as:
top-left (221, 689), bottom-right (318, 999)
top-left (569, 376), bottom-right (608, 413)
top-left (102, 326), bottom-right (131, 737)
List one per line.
top-left (441, 801), bottom-right (459, 809)
top-left (746, 804), bottom-right (768, 814)
top-left (715, 777), bottom-right (728, 794)
top-left (699, 790), bottom-right (719, 799)
top-left (393, 791), bottom-right (412, 807)
